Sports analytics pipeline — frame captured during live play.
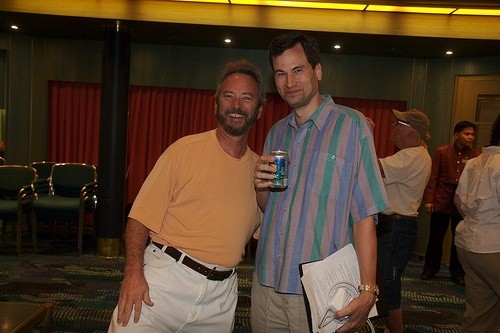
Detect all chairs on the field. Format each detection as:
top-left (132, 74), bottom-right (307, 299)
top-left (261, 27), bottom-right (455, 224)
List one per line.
top-left (0, 156), bottom-right (97, 259)
top-left (0, 301), bottom-right (53, 333)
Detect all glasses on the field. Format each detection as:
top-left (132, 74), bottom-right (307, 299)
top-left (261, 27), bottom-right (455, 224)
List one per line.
top-left (393, 120), bottom-right (410, 126)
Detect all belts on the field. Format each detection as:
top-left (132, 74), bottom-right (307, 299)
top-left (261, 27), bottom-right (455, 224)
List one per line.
top-left (151, 241), bottom-right (237, 281)
top-left (383, 215), bottom-right (417, 220)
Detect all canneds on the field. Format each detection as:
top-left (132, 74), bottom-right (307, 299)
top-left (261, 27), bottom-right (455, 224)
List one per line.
top-left (269, 151), bottom-right (288, 190)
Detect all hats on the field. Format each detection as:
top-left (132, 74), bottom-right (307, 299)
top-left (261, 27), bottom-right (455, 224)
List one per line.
top-left (392, 108), bottom-right (431, 140)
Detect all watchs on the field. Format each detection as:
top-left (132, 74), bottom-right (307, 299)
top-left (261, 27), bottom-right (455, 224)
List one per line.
top-left (358, 284), bottom-right (379, 296)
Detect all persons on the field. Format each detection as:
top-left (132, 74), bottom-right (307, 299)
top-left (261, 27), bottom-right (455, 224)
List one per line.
top-left (107, 59), bottom-right (267, 332)
top-left (420, 121), bottom-right (482, 287)
top-left (251, 33), bottom-right (390, 332)
top-left (454, 116), bottom-right (499, 332)
top-left (363, 108), bottom-right (432, 333)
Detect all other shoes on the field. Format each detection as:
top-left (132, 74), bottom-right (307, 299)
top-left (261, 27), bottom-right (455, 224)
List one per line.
top-left (419, 270), bottom-right (436, 280)
top-left (451, 276), bottom-right (464, 285)
